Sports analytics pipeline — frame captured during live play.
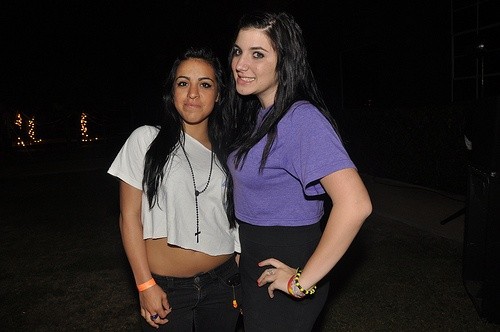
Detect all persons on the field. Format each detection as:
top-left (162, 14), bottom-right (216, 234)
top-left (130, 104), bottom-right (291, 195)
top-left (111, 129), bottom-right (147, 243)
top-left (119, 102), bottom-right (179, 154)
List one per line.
top-left (108, 48), bottom-right (242, 332)
top-left (226, 9), bottom-right (372, 332)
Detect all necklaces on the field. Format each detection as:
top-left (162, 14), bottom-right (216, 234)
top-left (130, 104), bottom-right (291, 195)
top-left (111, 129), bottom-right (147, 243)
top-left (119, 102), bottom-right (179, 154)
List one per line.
top-left (179, 138), bottom-right (214, 242)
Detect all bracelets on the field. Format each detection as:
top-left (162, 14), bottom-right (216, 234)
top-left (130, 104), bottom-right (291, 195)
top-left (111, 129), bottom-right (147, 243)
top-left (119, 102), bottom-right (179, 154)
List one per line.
top-left (287, 269), bottom-right (316, 298)
top-left (136, 278), bottom-right (157, 291)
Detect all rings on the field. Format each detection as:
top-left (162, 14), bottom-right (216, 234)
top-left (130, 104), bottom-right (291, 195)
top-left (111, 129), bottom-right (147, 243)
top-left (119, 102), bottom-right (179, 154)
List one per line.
top-left (151, 313), bottom-right (158, 320)
